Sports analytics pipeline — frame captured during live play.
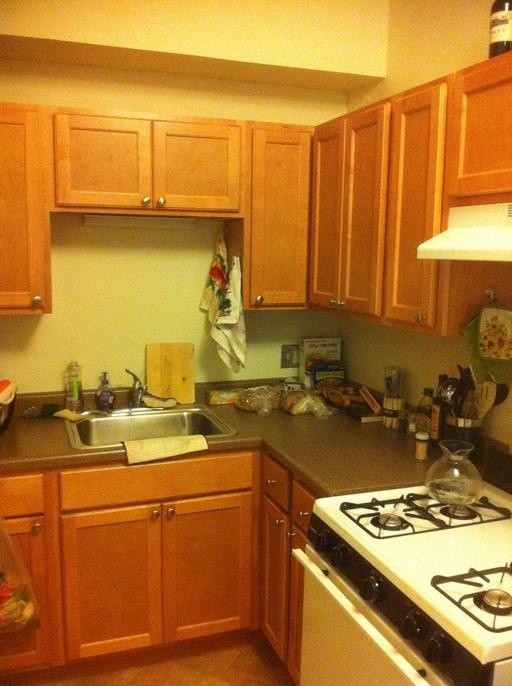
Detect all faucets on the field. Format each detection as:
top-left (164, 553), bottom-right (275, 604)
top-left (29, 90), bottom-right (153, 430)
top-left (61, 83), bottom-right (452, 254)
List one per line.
top-left (124, 368), bottom-right (146, 407)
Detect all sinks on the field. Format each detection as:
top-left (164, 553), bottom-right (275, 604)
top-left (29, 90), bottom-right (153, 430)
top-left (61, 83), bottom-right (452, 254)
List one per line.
top-left (65, 404), bottom-right (238, 450)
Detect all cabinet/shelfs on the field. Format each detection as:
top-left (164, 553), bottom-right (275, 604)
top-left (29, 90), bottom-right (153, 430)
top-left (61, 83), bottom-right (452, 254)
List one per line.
top-left (0, 472), bottom-right (50, 679)
top-left (258, 453), bottom-right (315, 684)
top-left (380, 81), bottom-right (448, 331)
top-left (448, 47), bottom-right (511, 202)
top-left (57, 452), bottom-right (252, 662)
top-left (0, 104), bottom-right (48, 315)
top-left (52, 112), bottom-right (242, 213)
top-left (248, 126), bottom-right (310, 309)
top-left (307, 101), bottom-right (390, 319)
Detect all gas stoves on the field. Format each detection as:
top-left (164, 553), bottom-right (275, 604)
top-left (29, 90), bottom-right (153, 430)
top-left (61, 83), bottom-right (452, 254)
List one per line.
top-left (305, 479), bottom-right (511, 686)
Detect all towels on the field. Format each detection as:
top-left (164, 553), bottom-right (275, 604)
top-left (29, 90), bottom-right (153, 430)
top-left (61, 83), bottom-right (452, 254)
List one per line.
top-left (121, 432), bottom-right (209, 467)
top-left (197, 226), bottom-right (246, 375)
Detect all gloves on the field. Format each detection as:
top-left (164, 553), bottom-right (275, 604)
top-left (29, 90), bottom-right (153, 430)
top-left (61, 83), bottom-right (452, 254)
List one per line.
top-left (463, 314), bottom-right (512, 384)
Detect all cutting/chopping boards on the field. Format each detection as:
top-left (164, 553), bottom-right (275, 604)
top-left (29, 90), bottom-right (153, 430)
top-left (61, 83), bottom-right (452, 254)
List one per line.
top-left (145, 343), bottom-right (194, 404)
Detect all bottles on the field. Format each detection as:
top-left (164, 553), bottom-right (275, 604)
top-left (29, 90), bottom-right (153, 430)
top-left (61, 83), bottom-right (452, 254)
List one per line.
top-left (414, 432), bottom-right (430, 460)
top-left (64, 361), bottom-right (85, 414)
top-left (417, 388), bottom-right (434, 418)
top-left (488, 0), bottom-right (512, 58)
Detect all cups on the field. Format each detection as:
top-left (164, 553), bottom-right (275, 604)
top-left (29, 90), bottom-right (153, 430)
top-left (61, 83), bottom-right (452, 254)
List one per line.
top-left (441, 413), bottom-right (482, 447)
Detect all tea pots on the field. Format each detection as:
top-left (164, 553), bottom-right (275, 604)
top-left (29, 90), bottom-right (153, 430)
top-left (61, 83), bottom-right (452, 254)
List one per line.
top-left (425, 439), bottom-right (484, 505)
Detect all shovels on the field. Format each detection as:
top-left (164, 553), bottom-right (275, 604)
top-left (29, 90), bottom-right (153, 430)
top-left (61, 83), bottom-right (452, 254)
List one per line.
top-left (477, 380), bottom-right (497, 420)
